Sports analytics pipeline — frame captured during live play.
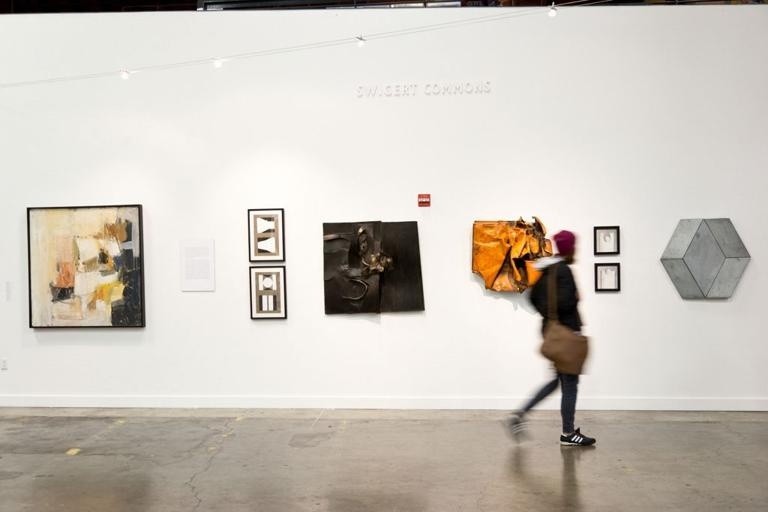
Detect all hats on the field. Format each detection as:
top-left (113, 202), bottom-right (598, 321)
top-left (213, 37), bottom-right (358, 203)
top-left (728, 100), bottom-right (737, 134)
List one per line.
top-left (552, 229), bottom-right (576, 256)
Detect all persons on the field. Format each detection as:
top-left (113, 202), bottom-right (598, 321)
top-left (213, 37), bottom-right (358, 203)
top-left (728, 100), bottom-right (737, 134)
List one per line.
top-left (499, 230), bottom-right (597, 448)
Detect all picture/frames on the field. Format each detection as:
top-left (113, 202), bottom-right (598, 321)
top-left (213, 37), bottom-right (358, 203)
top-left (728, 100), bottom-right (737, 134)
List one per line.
top-left (594, 263), bottom-right (621, 292)
top-left (248, 208), bottom-right (287, 263)
top-left (593, 226), bottom-right (621, 255)
top-left (248, 266), bottom-right (289, 321)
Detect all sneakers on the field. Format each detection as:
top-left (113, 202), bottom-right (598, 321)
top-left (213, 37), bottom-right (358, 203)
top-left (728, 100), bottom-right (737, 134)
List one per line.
top-left (504, 413), bottom-right (525, 444)
top-left (559, 427), bottom-right (596, 446)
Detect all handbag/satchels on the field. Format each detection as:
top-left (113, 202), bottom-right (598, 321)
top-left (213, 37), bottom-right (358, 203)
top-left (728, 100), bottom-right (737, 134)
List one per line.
top-left (539, 318), bottom-right (590, 376)
